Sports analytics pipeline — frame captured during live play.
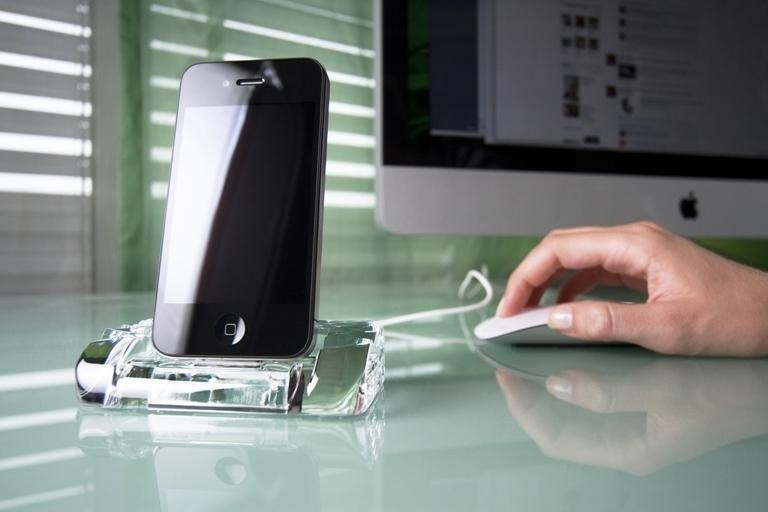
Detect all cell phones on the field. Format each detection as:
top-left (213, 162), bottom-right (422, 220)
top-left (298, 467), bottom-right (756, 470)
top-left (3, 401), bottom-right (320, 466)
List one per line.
top-left (152, 58), bottom-right (330, 359)
top-left (153, 446), bottom-right (318, 510)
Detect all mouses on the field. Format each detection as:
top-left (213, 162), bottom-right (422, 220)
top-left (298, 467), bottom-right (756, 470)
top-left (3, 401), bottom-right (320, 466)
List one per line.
top-left (473, 305), bottom-right (592, 346)
top-left (472, 347), bottom-right (648, 384)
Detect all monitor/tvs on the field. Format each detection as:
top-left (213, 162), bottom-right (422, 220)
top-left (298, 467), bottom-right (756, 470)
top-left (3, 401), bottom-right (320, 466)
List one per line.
top-left (373, 0), bottom-right (768, 239)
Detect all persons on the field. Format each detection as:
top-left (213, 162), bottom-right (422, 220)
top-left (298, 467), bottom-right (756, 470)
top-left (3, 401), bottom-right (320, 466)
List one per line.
top-left (495, 359), bottom-right (768, 476)
top-left (495, 220), bottom-right (768, 356)
